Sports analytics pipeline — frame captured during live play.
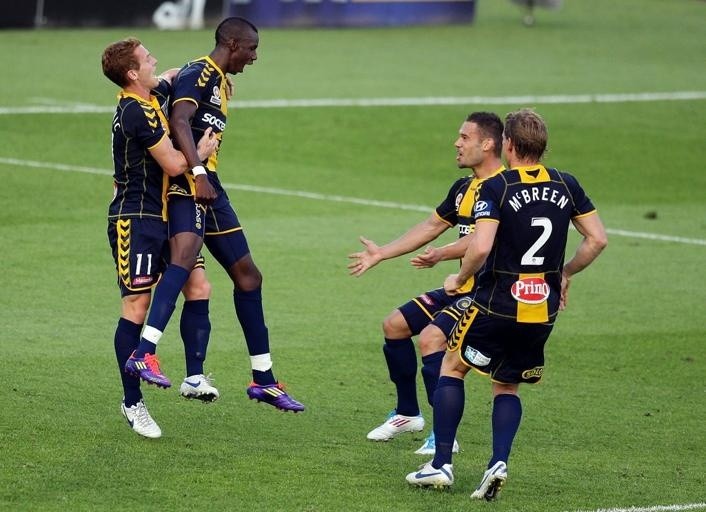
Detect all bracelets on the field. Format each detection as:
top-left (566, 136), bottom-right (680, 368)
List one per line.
top-left (190, 166), bottom-right (208, 178)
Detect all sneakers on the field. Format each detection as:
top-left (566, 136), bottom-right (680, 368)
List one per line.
top-left (247, 379), bottom-right (304, 413)
top-left (366, 409), bottom-right (507, 501)
top-left (121, 350), bottom-right (219, 439)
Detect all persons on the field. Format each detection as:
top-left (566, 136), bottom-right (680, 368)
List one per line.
top-left (345, 110), bottom-right (506, 457)
top-left (100, 38), bottom-right (235, 440)
top-left (124, 18), bottom-right (306, 413)
top-left (404, 107), bottom-right (608, 502)
